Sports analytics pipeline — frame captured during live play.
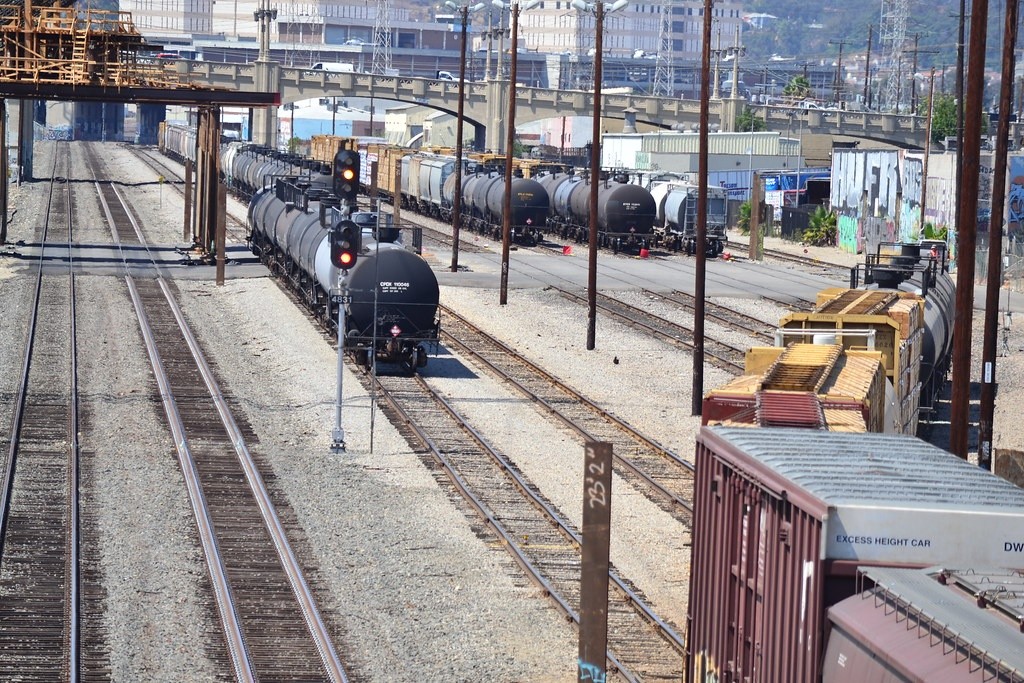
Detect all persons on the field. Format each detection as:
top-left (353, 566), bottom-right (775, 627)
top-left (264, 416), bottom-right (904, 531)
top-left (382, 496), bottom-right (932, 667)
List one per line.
top-left (927, 244), bottom-right (940, 262)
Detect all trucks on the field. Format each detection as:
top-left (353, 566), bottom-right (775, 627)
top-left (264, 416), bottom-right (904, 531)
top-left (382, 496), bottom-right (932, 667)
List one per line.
top-left (304, 62), bottom-right (354, 78)
top-left (427, 70), bottom-right (470, 89)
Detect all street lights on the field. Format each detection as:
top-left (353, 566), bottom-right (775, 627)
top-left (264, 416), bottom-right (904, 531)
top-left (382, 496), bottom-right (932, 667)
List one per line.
top-left (445, 1), bottom-right (486, 273)
top-left (571, 0), bottom-right (630, 350)
top-left (911, 65), bottom-right (936, 239)
top-left (492, 0), bottom-right (541, 305)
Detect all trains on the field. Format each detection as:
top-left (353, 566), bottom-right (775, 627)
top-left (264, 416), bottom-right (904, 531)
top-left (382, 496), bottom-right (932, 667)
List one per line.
top-left (157, 121), bottom-right (333, 207)
top-left (421, 142), bottom-right (665, 258)
top-left (246, 174), bottom-right (443, 376)
top-left (581, 162), bottom-right (730, 258)
top-left (311, 134), bottom-right (552, 247)
top-left (681, 238), bottom-right (1024, 683)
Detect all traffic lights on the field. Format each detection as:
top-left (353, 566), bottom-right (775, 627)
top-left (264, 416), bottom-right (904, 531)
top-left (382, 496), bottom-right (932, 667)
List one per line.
top-left (334, 221), bottom-right (358, 269)
top-left (334, 150), bottom-right (361, 199)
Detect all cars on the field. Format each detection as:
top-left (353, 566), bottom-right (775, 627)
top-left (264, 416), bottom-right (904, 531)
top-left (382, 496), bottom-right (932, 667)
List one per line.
top-left (766, 94), bottom-right (880, 120)
top-left (155, 53), bottom-right (185, 65)
top-left (118, 51), bottom-right (158, 64)
